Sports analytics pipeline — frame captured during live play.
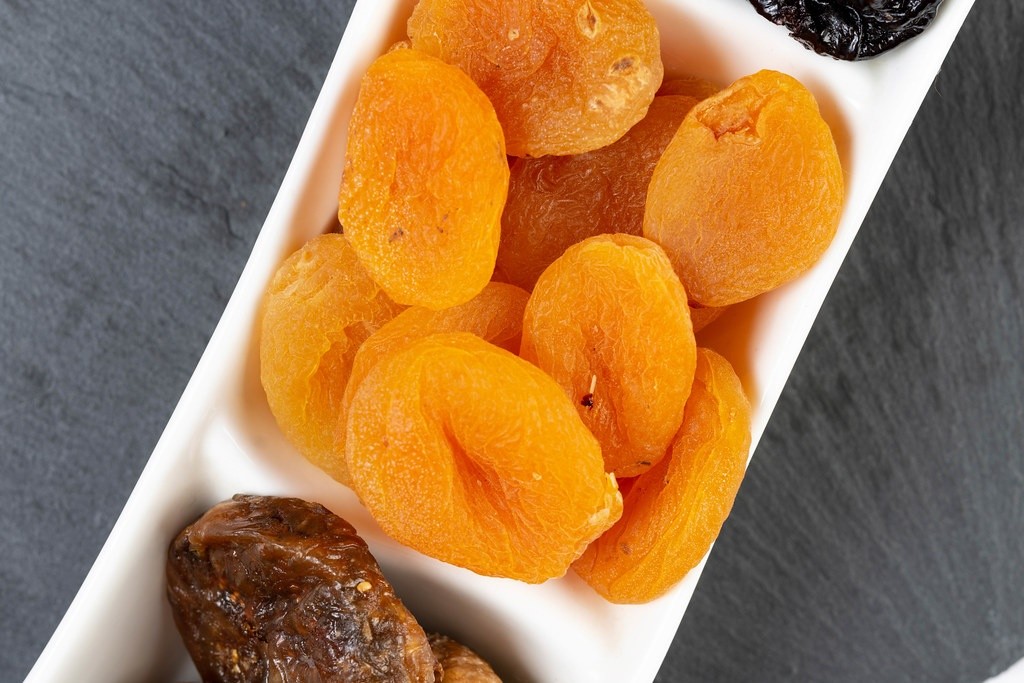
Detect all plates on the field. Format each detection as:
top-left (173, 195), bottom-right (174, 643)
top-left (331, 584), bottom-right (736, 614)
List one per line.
top-left (16, 0), bottom-right (981, 683)
